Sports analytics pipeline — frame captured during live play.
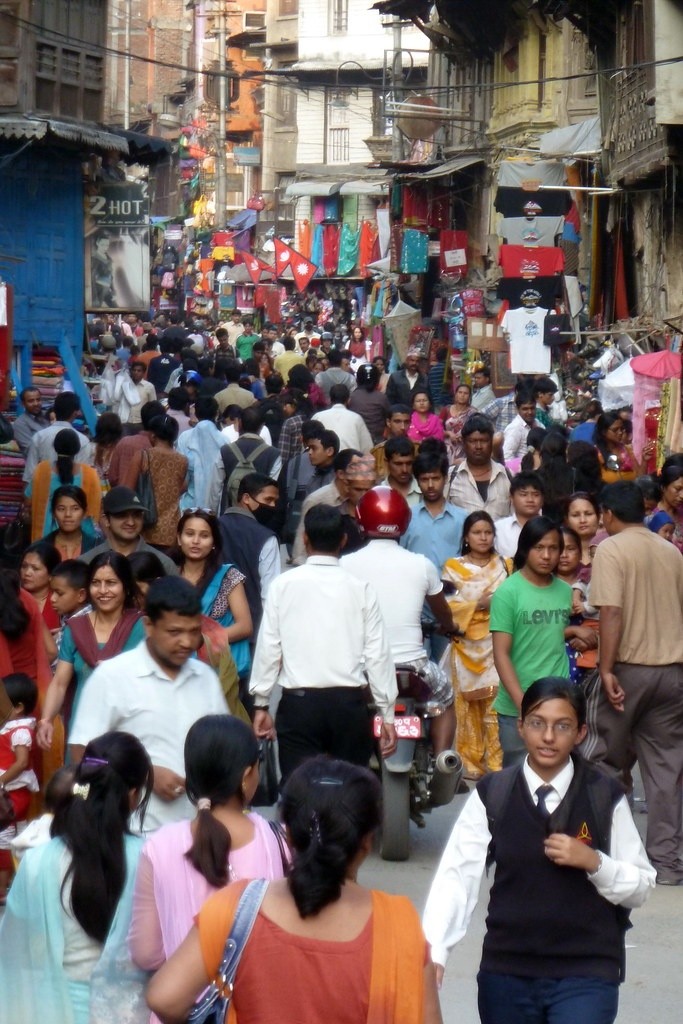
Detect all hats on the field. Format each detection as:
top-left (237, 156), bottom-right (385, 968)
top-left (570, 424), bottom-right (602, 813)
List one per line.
top-left (304, 317), bottom-right (313, 322)
top-left (589, 532), bottom-right (610, 547)
top-left (103, 486), bottom-right (150, 512)
top-left (102, 336), bottom-right (117, 348)
top-left (279, 388), bottom-right (308, 406)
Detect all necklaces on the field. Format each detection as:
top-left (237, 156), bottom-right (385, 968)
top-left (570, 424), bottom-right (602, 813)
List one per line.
top-left (39, 594), bottom-right (48, 602)
top-left (93, 611), bottom-right (98, 630)
top-left (471, 552), bottom-right (490, 560)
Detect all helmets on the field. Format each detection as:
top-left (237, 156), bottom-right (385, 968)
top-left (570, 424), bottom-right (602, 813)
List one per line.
top-left (321, 332), bottom-right (333, 339)
top-left (178, 370), bottom-right (202, 384)
top-left (355, 485), bottom-right (411, 538)
top-left (357, 365), bottom-right (379, 383)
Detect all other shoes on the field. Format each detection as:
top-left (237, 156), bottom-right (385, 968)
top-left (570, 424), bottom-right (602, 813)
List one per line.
top-left (656, 878), bottom-right (677, 884)
top-left (427, 780), bottom-right (470, 793)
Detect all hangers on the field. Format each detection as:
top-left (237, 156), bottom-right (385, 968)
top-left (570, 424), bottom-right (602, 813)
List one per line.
top-left (525, 157), bottom-right (537, 312)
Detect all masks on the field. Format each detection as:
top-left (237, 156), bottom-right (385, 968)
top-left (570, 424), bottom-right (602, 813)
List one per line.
top-left (247, 495), bottom-right (280, 531)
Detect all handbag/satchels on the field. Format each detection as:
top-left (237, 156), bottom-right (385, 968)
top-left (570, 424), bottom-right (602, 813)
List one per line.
top-left (99, 353), bottom-right (140, 424)
top-left (251, 737), bottom-right (278, 806)
top-left (282, 454), bottom-right (303, 540)
top-left (543, 306), bottom-right (574, 345)
top-left (137, 449), bottom-right (157, 529)
top-left (186, 879), bottom-right (270, 1024)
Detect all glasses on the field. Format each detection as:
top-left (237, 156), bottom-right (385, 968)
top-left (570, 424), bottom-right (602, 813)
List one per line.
top-left (608, 428), bottom-right (626, 432)
top-left (185, 507), bottom-right (217, 516)
top-left (525, 719), bottom-right (579, 736)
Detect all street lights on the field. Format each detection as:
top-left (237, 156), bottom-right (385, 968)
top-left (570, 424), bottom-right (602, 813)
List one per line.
top-left (158, 113), bottom-right (227, 231)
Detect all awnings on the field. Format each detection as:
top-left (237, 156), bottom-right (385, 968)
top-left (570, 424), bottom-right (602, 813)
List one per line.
top-left (365, 157), bottom-right (485, 179)
top-left (243, 55), bottom-right (429, 78)
top-left (286, 180), bottom-right (391, 196)
top-left (226, 30), bottom-right (266, 49)
top-left (0, 111), bottom-right (182, 183)
top-left (250, 39), bottom-right (298, 49)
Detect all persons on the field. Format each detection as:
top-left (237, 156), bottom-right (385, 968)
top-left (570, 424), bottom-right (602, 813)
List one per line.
top-left (0, 731), bottom-right (156, 1024)
top-left (0, 242), bottom-right (683, 904)
top-left (421, 676), bottom-right (657, 1024)
top-left (130, 715), bottom-right (297, 1024)
top-left (145, 758), bottom-right (444, 1024)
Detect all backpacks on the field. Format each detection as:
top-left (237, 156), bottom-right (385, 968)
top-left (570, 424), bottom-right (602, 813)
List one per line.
top-left (229, 442), bottom-right (270, 507)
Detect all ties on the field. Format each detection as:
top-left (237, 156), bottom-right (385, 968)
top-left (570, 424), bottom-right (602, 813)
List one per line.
top-left (535, 785), bottom-right (553, 823)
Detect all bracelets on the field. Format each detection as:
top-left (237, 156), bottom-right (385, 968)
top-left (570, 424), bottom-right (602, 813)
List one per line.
top-left (254, 705), bottom-right (269, 710)
top-left (38, 719), bottom-right (52, 728)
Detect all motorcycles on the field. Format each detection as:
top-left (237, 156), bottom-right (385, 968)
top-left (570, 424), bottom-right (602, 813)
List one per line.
top-left (367, 579), bottom-right (463, 862)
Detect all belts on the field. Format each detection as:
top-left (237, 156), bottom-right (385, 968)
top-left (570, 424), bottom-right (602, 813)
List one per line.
top-left (283, 687), bottom-right (369, 701)
